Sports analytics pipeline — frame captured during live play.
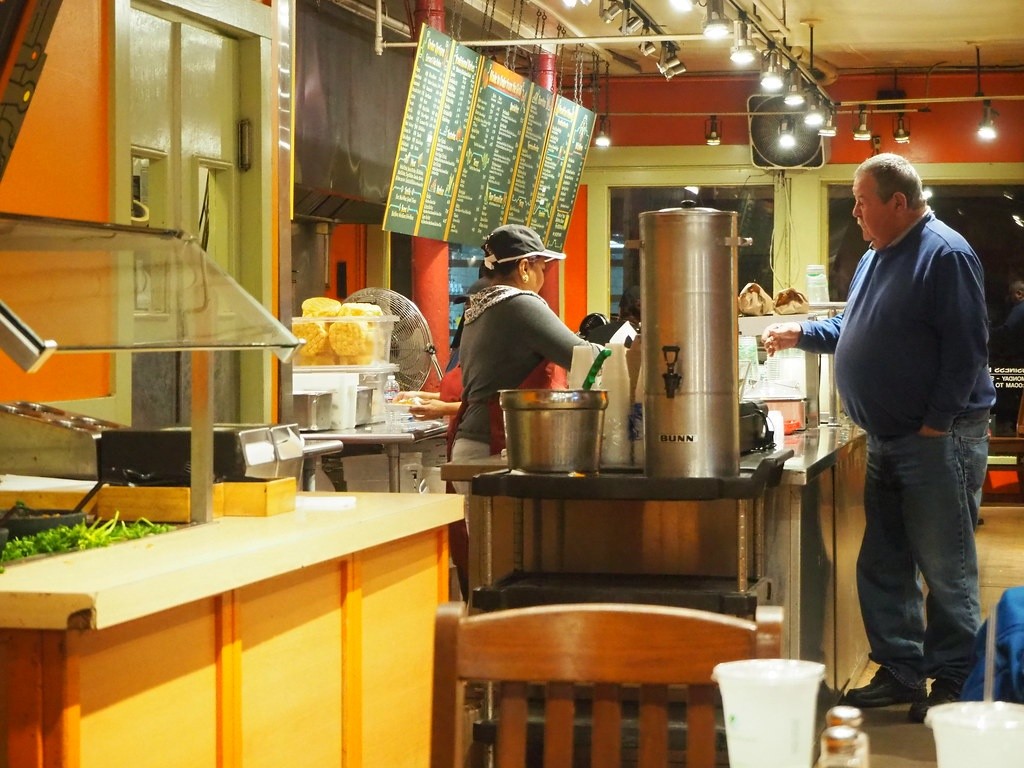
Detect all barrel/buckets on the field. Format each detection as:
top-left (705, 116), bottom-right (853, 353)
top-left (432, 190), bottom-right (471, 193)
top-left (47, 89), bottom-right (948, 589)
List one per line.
top-left (496, 387), bottom-right (607, 473)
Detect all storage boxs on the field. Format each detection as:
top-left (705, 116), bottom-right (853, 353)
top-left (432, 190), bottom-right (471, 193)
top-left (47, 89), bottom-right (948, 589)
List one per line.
top-left (291, 316), bottom-right (400, 429)
top-left (0, 473), bottom-right (297, 523)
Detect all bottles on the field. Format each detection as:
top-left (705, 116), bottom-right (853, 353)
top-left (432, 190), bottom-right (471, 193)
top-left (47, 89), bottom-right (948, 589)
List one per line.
top-left (384, 375), bottom-right (400, 402)
top-left (814, 705), bottom-right (869, 768)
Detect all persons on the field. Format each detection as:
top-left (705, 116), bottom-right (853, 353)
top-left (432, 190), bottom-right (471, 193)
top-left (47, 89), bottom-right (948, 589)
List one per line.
top-left (761, 153), bottom-right (995, 723)
top-left (450, 224), bottom-right (609, 495)
top-left (588, 287), bottom-right (641, 346)
top-left (392, 276), bottom-right (492, 430)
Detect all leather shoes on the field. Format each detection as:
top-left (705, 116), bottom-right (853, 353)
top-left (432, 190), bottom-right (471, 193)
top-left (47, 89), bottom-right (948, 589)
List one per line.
top-left (845, 667), bottom-right (926, 707)
top-left (907, 684), bottom-right (960, 724)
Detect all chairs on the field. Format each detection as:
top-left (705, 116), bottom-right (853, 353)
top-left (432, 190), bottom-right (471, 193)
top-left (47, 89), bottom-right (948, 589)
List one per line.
top-left (982, 391), bottom-right (1024, 506)
top-left (419, 601), bottom-right (784, 768)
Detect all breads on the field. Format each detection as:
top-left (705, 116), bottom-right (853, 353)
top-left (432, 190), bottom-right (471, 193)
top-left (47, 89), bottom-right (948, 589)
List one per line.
top-left (287, 295), bottom-right (387, 367)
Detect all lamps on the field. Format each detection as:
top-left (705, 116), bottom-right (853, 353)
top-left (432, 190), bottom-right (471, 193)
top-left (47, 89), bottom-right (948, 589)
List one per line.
top-left (558, 0), bottom-right (999, 148)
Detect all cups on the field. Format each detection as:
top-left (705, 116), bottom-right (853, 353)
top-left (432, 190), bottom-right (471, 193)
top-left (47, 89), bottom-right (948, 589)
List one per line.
top-left (713, 659), bottom-right (824, 768)
top-left (569, 344), bottom-right (645, 470)
top-left (927, 701), bottom-right (1024, 768)
top-left (385, 403), bottom-right (415, 434)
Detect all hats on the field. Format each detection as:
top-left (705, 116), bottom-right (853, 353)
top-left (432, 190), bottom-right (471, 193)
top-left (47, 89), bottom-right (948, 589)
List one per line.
top-left (620, 285), bottom-right (641, 314)
top-left (482, 223), bottom-right (566, 270)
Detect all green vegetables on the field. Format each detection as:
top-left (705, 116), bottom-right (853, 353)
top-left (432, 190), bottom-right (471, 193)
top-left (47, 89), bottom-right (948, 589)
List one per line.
top-left (0, 510), bottom-right (178, 574)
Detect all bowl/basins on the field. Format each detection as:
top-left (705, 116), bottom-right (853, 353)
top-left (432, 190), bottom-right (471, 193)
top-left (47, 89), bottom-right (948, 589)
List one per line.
top-left (0, 508), bottom-right (87, 541)
